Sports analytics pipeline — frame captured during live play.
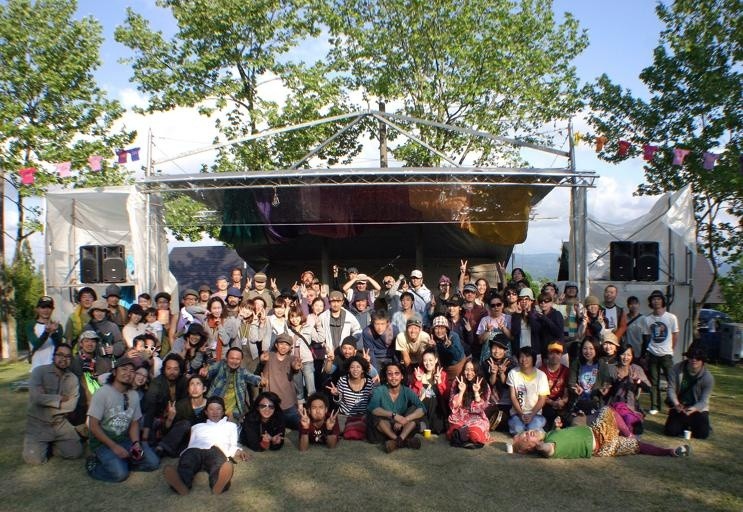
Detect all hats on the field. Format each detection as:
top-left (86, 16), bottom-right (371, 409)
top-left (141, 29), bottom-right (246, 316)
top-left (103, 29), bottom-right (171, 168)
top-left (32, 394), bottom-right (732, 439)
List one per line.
top-left (446, 294), bottom-right (463, 306)
top-left (198, 284), bottom-right (211, 291)
top-left (128, 303), bottom-right (144, 314)
top-left (681, 341), bottom-right (710, 362)
top-left (519, 287), bottom-right (536, 301)
top-left (87, 300), bottom-right (111, 314)
top-left (586, 295), bottom-right (600, 305)
top-left (648, 289), bottom-right (665, 299)
top-left (410, 269), bottom-right (423, 279)
top-left (547, 340), bottom-right (564, 353)
top-left (356, 273), bottom-right (368, 284)
top-left (354, 292), bottom-right (367, 300)
top-left (102, 284), bottom-right (124, 299)
top-left (463, 285), bottom-right (478, 293)
top-left (437, 274), bottom-right (453, 289)
top-left (488, 333), bottom-right (510, 350)
top-left (277, 333), bottom-right (293, 345)
top-left (430, 315), bottom-right (450, 330)
top-left (599, 332), bottom-right (621, 348)
top-left (183, 289), bottom-right (199, 298)
top-left (253, 272), bottom-right (268, 282)
top-left (329, 291), bottom-right (344, 301)
top-left (405, 315), bottom-right (422, 328)
top-left (79, 330), bottom-right (100, 341)
top-left (225, 287), bottom-right (243, 301)
top-left (186, 323), bottom-right (208, 337)
top-left (115, 357), bottom-right (135, 368)
top-left (341, 336), bottom-right (357, 349)
top-left (563, 282), bottom-right (578, 294)
top-left (348, 267), bottom-right (358, 273)
top-left (155, 292), bottom-right (171, 301)
top-left (37, 296), bottom-right (54, 309)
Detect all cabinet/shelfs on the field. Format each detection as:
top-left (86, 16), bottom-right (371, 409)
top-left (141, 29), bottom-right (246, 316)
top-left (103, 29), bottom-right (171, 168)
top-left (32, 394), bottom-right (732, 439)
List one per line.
top-left (718, 322), bottom-right (743, 361)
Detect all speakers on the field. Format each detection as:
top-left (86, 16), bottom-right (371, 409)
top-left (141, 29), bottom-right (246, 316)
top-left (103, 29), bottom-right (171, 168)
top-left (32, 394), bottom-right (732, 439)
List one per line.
top-left (102, 244), bottom-right (126, 282)
top-left (80, 245), bottom-right (102, 283)
top-left (610, 241), bottom-right (633, 281)
top-left (634, 241), bottom-right (659, 281)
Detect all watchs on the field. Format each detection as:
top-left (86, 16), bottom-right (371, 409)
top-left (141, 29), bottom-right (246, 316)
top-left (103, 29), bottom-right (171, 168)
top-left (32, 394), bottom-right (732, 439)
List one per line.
top-left (392, 411), bottom-right (397, 419)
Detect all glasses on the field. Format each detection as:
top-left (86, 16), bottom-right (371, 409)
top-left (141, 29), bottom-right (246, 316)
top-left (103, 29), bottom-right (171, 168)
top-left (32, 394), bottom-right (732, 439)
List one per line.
top-left (505, 292), bottom-right (516, 296)
top-left (407, 319), bottom-right (421, 324)
top-left (259, 403), bottom-right (274, 409)
top-left (687, 355), bottom-right (701, 362)
top-left (54, 352), bottom-right (73, 360)
top-left (185, 298), bottom-right (197, 302)
top-left (144, 345), bottom-right (155, 351)
top-left (284, 296), bottom-right (292, 300)
top-left (466, 290), bottom-right (475, 295)
top-left (490, 303), bottom-right (502, 308)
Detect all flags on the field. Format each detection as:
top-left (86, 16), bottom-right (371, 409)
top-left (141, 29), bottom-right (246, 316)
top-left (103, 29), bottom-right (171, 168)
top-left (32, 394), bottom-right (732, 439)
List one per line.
top-left (574, 129), bottom-right (718, 171)
top-left (20, 146), bottom-right (141, 186)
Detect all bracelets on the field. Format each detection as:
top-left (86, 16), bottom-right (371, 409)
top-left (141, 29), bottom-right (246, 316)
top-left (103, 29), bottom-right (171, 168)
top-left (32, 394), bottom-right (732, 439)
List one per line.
top-left (132, 440), bottom-right (139, 446)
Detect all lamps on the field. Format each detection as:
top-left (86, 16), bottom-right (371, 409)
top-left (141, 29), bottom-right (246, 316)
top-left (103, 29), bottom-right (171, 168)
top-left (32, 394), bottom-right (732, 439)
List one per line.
top-left (272, 188), bottom-right (280, 205)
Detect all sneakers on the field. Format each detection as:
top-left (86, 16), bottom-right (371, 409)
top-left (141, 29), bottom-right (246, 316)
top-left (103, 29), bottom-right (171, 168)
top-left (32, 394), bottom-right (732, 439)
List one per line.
top-left (385, 440), bottom-right (398, 451)
top-left (450, 428), bottom-right (462, 446)
top-left (464, 442), bottom-right (484, 450)
top-left (163, 464), bottom-right (189, 495)
top-left (212, 461), bottom-right (233, 494)
top-left (405, 436), bottom-right (422, 450)
top-left (674, 445), bottom-right (691, 456)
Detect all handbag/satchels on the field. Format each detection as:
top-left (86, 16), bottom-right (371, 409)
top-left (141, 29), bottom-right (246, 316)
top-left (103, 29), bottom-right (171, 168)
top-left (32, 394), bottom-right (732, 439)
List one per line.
top-left (289, 326), bottom-right (324, 359)
top-left (343, 416), bottom-right (367, 439)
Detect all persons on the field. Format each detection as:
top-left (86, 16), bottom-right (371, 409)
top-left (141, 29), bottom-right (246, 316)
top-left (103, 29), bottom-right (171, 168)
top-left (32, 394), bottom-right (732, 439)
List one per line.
top-left (163, 396), bottom-right (248, 495)
top-left (281, 288), bottom-right (292, 310)
top-left (143, 307), bottom-right (162, 347)
top-left (196, 285), bottom-right (213, 309)
top-left (68, 330), bottom-right (113, 385)
top-left (254, 296), bottom-right (267, 315)
top-left (292, 270), bottom-right (314, 299)
top-left (363, 309), bottom-right (399, 384)
top-left (132, 336), bottom-right (145, 352)
top-left (512, 406), bottom-right (689, 457)
top-left (286, 305), bottom-right (325, 397)
top-left (85, 358), bottom-right (159, 482)
top-left (447, 358), bottom-right (495, 450)
top-left (173, 323), bottom-right (209, 369)
top-left (478, 260), bottom-right (550, 434)
top-left (122, 304), bottom-right (160, 348)
top-left (227, 268), bottom-right (244, 293)
top-left (142, 353), bottom-right (189, 438)
top-left (131, 365), bottom-right (148, 405)
top-left (187, 297), bottom-right (233, 359)
top-left (231, 300), bottom-right (261, 373)
top-left (210, 275), bottom-right (229, 301)
top-left (242, 272), bottom-right (281, 310)
top-left (154, 292), bottom-right (173, 328)
top-left (199, 347), bottom-right (267, 438)
top-left (298, 392), bottom-right (340, 451)
top-left (224, 287), bottom-right (244, 318)
top-left (183, 289), bottom-right (200, 306)
top-left (326, 356), bottom-right (380, 439)
top-left (347, 267), bottom-right (358, 280)
top-left (534, 280), bottom-right (680, 416)
top-left (306, 297), bottom-right (326, 373)
top-left (63, 287), bottom-right (97, 356)
top-left (123, 334), bottom-right (163, 378)
top-left (101, 285), bottom-right (129, 330)
top-left (408, 348), bottom-right (449, 436)
top-left (343, 274), bottom-right (381, 307)
top-left (259, 298), bottom-right (286, 352)
top-left (138, 293), bottom-right (152, 310)
top-left (319, 291), bottom-right (363, 353)
top-left (156, 374), bottom-right (208, 460)
top-left (665, 341), bottom-right (715, 440)
top-left (367, 362), bottom-right (426, 452)
top-left (333, 336), bottom-right (378, 379)
top-left (22, 344), bottom-right (82, 464)
top-left (240, 392), bottom-right (285, 452)
top-left (261, 334), bottom-right (303, 431)
top-left (82, 300), bottom-right (127, 357)
top-left (347, 291), bottom-right (373, 330)
top-left (382, 259), bottom-right (492, 362)
top-left (25, 296), bottom-right (63, 368)
top-left (300, 283), bottom-right (329, 314)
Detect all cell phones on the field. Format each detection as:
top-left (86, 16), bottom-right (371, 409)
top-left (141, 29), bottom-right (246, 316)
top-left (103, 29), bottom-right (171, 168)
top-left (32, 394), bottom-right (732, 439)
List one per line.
top-left (130, 448), bottom-right (144, 457)
